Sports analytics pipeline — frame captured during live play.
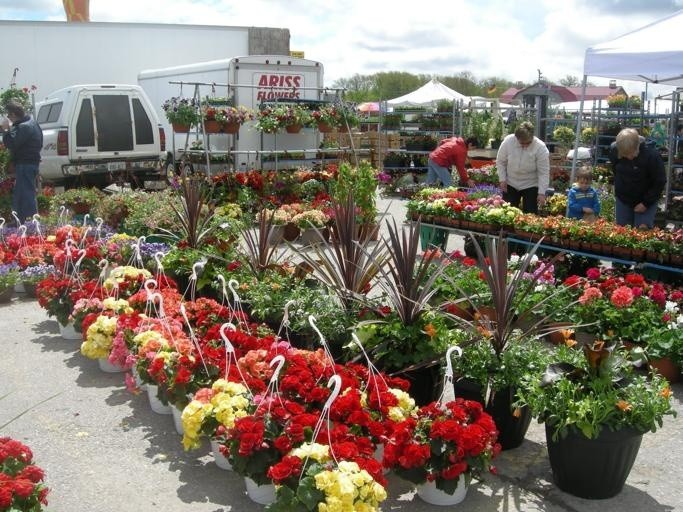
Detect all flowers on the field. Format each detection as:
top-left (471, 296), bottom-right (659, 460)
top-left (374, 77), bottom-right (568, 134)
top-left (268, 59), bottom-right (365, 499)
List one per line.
top-left (1, 158), bottom-right (683, 511)
top-left (161, 98), bottom-right (201, 112)
top-left (261, 105), bottom-right (294, 120)
top-left (204, 104), bottom-right (256, 123)
top-left (327, 105), bottom-right (337, 116)
top-left (311, 109), bottom-right (322, 119)
top-left (21, 84), bottom-right (37, 96)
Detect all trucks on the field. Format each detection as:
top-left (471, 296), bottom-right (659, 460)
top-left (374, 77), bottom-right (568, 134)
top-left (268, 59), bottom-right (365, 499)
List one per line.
top-left (137, 54), bottom-right (325, 177)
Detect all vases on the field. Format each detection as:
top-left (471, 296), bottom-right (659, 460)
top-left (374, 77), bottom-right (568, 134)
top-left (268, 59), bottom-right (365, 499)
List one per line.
top-left (204, 120), bottom-right (239, 133)
top-left (286, 125), bottom-right (303, 134)
top-left (318, 122), bottom-right (334, 133)
top-left (172, 122), bottom-right (190, 132)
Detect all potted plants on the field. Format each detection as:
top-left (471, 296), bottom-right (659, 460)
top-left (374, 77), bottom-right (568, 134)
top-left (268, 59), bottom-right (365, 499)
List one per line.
top-left (336, 108), bottom-right (359, 132)
top-left (463, 109), bottom-right (504, 150)
top-left (606, 93), bottom-right (642, 111)
top-left (360, 98), bottom-right (453, 151)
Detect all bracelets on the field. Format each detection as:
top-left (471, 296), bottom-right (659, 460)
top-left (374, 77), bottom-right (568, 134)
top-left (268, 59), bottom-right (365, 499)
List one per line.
top-left (4, 128), bottom-right (10, 131)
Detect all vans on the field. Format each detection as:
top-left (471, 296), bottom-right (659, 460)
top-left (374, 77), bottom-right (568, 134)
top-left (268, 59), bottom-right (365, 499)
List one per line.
top-left (32, 84), bottom-right (174, 195)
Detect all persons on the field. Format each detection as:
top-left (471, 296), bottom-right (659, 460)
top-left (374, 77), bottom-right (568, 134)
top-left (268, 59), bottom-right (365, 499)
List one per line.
top-left (428, 135), bottom-right (478, 188)
top-left (565, 168), bottom-right (600, 220)
top-left (495, 122), bottom-right (550, 215)
top-left (609, 128), bottom-right (667, 228)
top-left (2, 103), bottom-right (43, 223)
